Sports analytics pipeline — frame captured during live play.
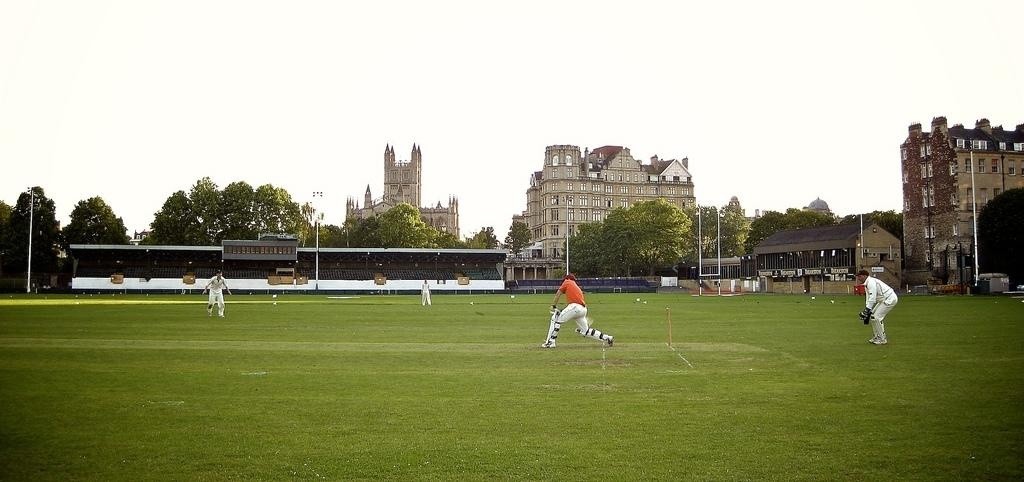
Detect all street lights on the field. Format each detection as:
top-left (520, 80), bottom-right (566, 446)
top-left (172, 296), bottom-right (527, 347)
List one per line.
top-left (562, 195), bottom-right (573, 278)
top-left (964, 135), bottom-right (979, 287)
top-left (27, 186), bottom-right (38, 294)
top-left (312, 191), bottom-right (324, 290)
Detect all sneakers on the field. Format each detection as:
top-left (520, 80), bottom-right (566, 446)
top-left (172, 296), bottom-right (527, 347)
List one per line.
top-left (869, 337), bottom-right (887, 345)
top-left (542, 341), bottom-right (556, 347)
top-left (608, 336), bottom-right (614, 346)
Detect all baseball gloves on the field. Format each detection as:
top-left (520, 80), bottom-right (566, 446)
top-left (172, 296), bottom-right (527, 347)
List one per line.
top-left (858, 308), bottom-right (870, 320)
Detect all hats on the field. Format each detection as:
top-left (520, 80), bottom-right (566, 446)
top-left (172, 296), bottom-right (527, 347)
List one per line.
top-left (564, 274), bottom-right (575, 279)
top-left (855, 270), bottom-right (869, 276)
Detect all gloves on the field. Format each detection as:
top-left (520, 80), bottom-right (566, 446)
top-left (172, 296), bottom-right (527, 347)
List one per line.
top-left (859, 307), bottom-right (873, 325)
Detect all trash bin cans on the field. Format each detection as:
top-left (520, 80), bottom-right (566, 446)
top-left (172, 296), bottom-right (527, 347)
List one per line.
top-left (914, 285), bottom-right (928, 296)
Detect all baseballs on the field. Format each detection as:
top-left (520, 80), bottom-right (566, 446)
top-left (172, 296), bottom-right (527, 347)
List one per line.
top-left (273, 295), bottom-right (276, 298)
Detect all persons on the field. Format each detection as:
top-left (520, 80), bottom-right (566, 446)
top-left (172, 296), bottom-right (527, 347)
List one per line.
top-left (202, 269), bottom-right (232, 319)
top-left (541, 274), bottom-right (615, 348)
top-left (420, 280), bottom-right (432, 307)
top-left (855, 269), bottom-right (899, 344)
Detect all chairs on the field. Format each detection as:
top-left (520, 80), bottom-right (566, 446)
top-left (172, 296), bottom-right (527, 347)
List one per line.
top-left (75, 263), bottom-right (501, 280)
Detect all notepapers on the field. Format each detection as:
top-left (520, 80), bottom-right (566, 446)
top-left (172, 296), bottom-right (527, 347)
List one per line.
top-left (853, 284), bottom-right (865, 296)
